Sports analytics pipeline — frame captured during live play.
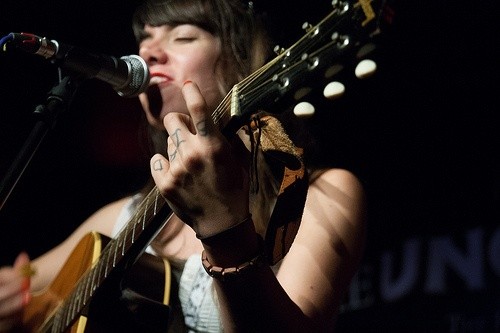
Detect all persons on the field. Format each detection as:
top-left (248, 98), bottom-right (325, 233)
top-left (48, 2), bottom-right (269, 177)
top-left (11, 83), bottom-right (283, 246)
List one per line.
top-left (0, 0), bottom-right (371, 333)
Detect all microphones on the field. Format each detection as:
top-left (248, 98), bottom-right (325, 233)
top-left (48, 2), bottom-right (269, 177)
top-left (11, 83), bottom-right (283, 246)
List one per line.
top-left (4, 32), bottom-right (151, 101)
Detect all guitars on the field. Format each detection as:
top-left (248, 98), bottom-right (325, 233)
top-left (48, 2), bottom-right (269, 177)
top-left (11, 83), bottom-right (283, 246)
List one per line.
top-left (22, 0), bottom-right (376, 333)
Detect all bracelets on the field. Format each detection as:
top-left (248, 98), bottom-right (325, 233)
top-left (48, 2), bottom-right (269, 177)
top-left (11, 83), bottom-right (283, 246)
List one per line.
top-left (201, 232), bottom-right (268, 284)
top-left (195, 210), bottom-right (253, 241)
